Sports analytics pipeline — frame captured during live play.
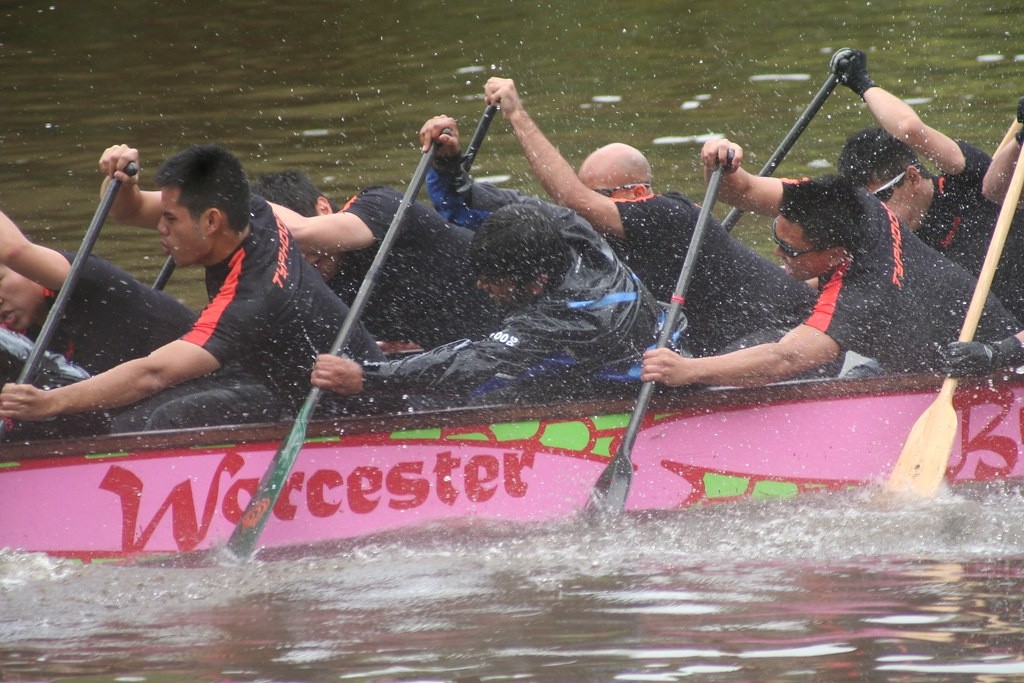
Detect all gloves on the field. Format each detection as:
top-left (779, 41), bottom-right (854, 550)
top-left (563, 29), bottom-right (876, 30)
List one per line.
top-left (938, 336), bottom-right (1024, 379)
top-left (1014, 95), bottom-right (1023, 149)
top-left (827, 45), bottom-right (880, 100)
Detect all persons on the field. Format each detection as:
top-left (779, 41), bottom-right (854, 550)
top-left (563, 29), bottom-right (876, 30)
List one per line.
top-left (0, 203), bottom-right (282, 437)
top-left (255, 46), bottom-right (1024, 399)
top-left (0, 143), bottom-right (396, 436)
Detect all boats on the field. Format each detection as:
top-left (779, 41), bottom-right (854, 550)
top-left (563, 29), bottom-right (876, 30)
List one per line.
top-left (0, 367), bottom-right (1024, 571)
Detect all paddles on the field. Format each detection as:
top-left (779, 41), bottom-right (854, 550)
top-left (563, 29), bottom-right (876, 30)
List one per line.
top-left (1, 157), bottom-right (141, 434)
top-left (212, 131), bottom-right (447, 554)
top-left (879, 142), bottom-right (1024, 497)
top-left (721, 60), bottom-right (845, 243)
top-left (461, 93), bottom-right (499, 179)
top-left (576, 152), bottom-right (735, 525)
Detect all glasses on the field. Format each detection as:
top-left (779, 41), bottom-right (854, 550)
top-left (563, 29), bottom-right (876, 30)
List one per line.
top-left (874, 160), bottom-right (924, 203)
top-left (592, 181), bottom-right (652, 198)
top-left (771, 218), bottom-right (831, 257)
